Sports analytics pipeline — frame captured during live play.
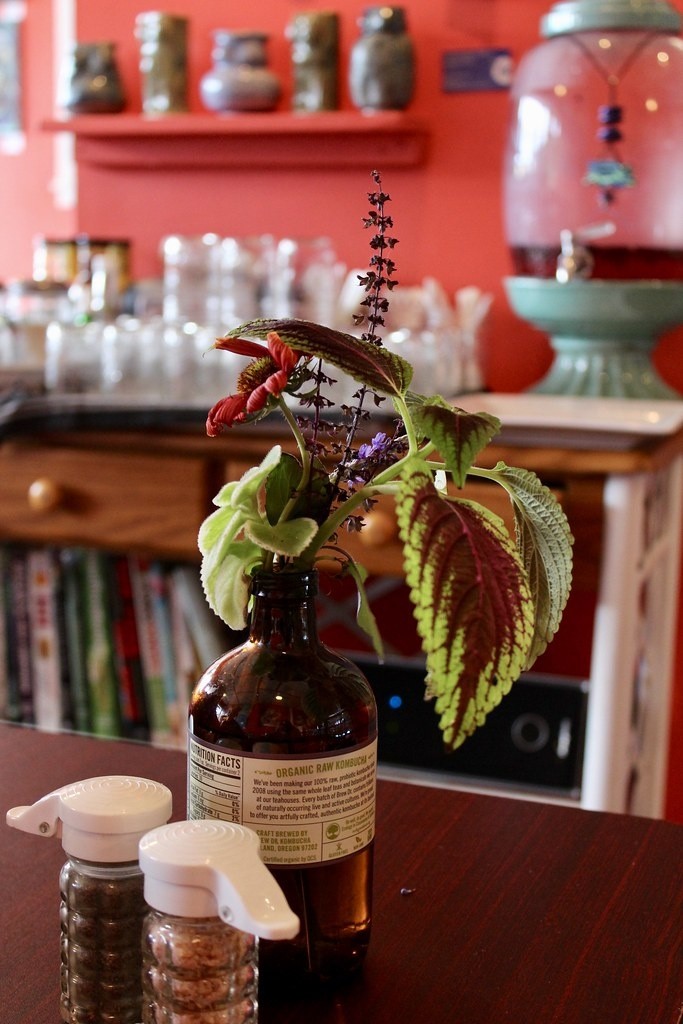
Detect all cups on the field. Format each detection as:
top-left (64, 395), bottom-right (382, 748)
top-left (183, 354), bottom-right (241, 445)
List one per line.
top-left (40, 236), bottom-right (461, 395)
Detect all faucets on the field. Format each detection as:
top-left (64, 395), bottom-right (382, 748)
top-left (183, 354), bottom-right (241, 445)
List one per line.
top-left (555, 222), bottom-right (616, 282)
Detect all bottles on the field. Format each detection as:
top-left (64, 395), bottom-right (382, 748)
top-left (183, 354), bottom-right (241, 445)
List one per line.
top-left (136, 820), bottom-right (299, 1023)
top-left (7, 775), bottom-right (173, 1024)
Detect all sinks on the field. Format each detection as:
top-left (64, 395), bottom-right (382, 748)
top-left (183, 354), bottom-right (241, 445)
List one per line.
top-left (502, 276), bottom-right (682, 340)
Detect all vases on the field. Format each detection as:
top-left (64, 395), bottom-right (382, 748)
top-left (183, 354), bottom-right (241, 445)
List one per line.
top-left (185, 563), bottom-right (377, 966)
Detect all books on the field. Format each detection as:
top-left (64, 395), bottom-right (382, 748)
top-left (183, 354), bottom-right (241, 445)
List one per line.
top-left (1, 536), bottom-right (227, 750)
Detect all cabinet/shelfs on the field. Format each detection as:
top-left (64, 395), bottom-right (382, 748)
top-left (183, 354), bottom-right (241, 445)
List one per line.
top-left (0, 0), bottom-right (683, 819)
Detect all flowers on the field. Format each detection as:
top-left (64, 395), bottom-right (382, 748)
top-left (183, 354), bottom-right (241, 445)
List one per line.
top-left (197, 169), bottom-right (575, 756)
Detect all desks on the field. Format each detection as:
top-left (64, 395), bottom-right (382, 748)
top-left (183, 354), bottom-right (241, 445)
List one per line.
top-left (0, 722), bottom-right (683, 1024)
